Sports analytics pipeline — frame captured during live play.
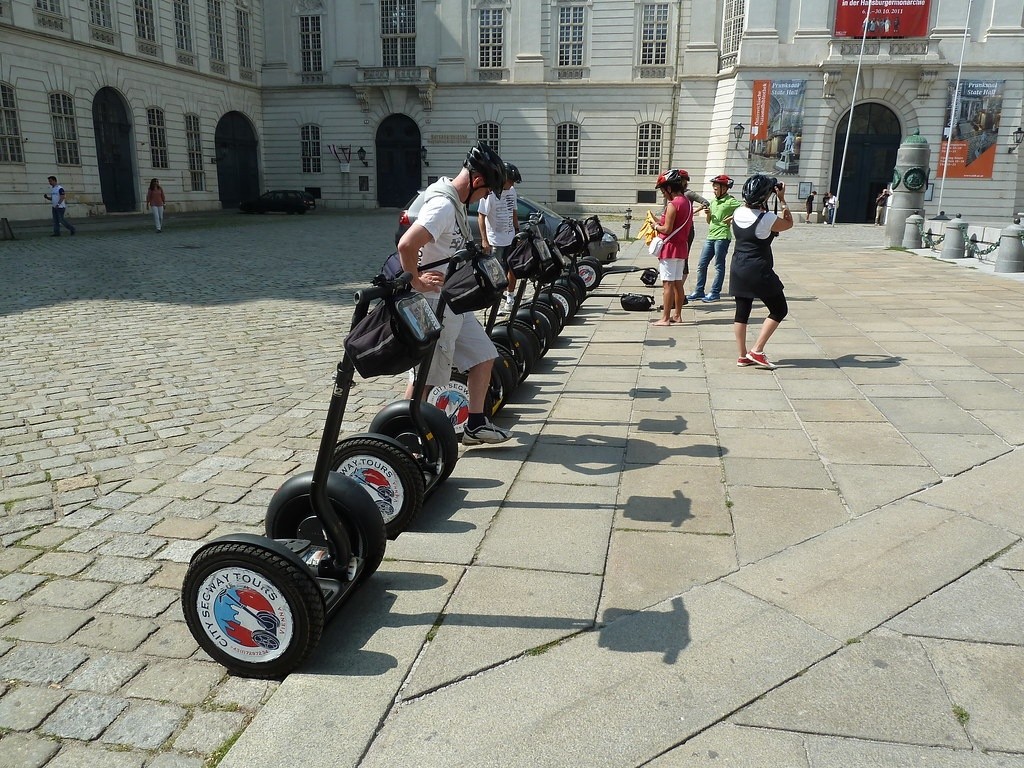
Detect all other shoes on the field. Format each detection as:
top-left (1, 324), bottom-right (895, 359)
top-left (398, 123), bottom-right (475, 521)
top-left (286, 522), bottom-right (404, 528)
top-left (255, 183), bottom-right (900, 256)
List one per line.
top-left (660, 296), bottom-right (688, 309)
top-left (70, 226), bottom-right (76, 236)
top-left (805, 221), bottom-right (812, 224)
top-left (462, 416), bottom-right (515, 446)
top-left (505, 291), bottom-right (514, 311)
top-left (156, 230), bottom-right (161, 233)
top-left (497, 299), bottom-right (505, 315)
top-left (687, 291), bottom-right (705, 301)
top-left (50, 232), bottom-right (61, 237)
top-left (702, 293), bottom-right (720, 303)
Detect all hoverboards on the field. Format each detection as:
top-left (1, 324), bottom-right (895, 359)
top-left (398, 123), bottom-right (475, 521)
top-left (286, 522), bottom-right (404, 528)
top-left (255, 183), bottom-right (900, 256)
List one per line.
top-left (180, 289), bottom-right (406, 679)
top-left (423, 216), bottom-right (603, 439)
top-left (323, 249), bottom-right (467, 533)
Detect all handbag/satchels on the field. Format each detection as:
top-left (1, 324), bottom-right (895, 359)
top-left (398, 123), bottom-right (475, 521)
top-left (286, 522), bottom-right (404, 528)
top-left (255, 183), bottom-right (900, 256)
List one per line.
top-left (554, 220), bottom-right (589, 255)
top-left (507, 236), bottom-right (555, 280)
top-left (343, 290), bottom-right (444, 378)
top-left (586, 219), bottom-right (604, 242)
top-left (621, 293), bottom-right (655, 311)
top-left (441, 251), bottom-right (510, 314)
top-left (648, 236), bottom-right (664, 257)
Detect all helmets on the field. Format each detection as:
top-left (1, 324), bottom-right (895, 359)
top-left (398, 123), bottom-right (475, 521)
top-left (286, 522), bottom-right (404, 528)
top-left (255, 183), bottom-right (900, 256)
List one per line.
top-left (463, 140), bottom-right (506, 200)
top-left (503, 162), bottom-right (522, 183)
top-left (710, 175), bottom-right (734, 189)
top-left (679, 169), bottom-right (690, 181)
top-left (742, 174), bottom-right (778, 208)
top-left (655, 169), bottom-right (681, 189)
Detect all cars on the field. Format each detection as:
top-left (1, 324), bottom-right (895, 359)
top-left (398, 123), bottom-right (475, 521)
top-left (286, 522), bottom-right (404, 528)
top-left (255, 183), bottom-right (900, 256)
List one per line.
top-left (238, 190), bottom-right (316, 215)
top-left (394, 191), bottom-right (620, 264)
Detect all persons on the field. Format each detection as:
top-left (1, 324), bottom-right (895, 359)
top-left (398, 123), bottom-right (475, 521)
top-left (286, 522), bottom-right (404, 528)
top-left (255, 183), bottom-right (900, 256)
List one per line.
top-left (44, 176), bottom-right (76, 236)
top-left (730, 174), bottom-right (793, 369)
top-left (649, 168), bottom-right (837, 326)
top-left (146, 178), bottom-right (166, 232)
top-left (397, 141), bottom-right (514, 445)
top-left (477, 162), bottom-right (523, 311)
top-left (875, 188), bottom-right (892, 226)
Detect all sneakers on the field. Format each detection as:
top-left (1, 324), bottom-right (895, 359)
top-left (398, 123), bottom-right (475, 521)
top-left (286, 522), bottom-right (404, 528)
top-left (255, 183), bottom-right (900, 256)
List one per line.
top-left (746, 350), bottom-right (775, 367)
top-left (737, 357), bottom-right (756, 366)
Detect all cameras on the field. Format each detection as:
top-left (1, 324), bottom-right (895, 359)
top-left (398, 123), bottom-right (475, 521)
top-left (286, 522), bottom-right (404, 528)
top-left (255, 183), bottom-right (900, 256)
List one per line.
top-left (771, 183), bottom-right (782, 193)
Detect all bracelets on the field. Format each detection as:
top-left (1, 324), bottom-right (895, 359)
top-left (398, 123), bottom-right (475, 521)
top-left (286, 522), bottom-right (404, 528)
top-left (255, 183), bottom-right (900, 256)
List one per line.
top-left (655, 225), bottom-right (658, 230)
top-left (57, 203), bottom-right (60, 205)
top-left (782, 204), bottom-right (788, 209)
